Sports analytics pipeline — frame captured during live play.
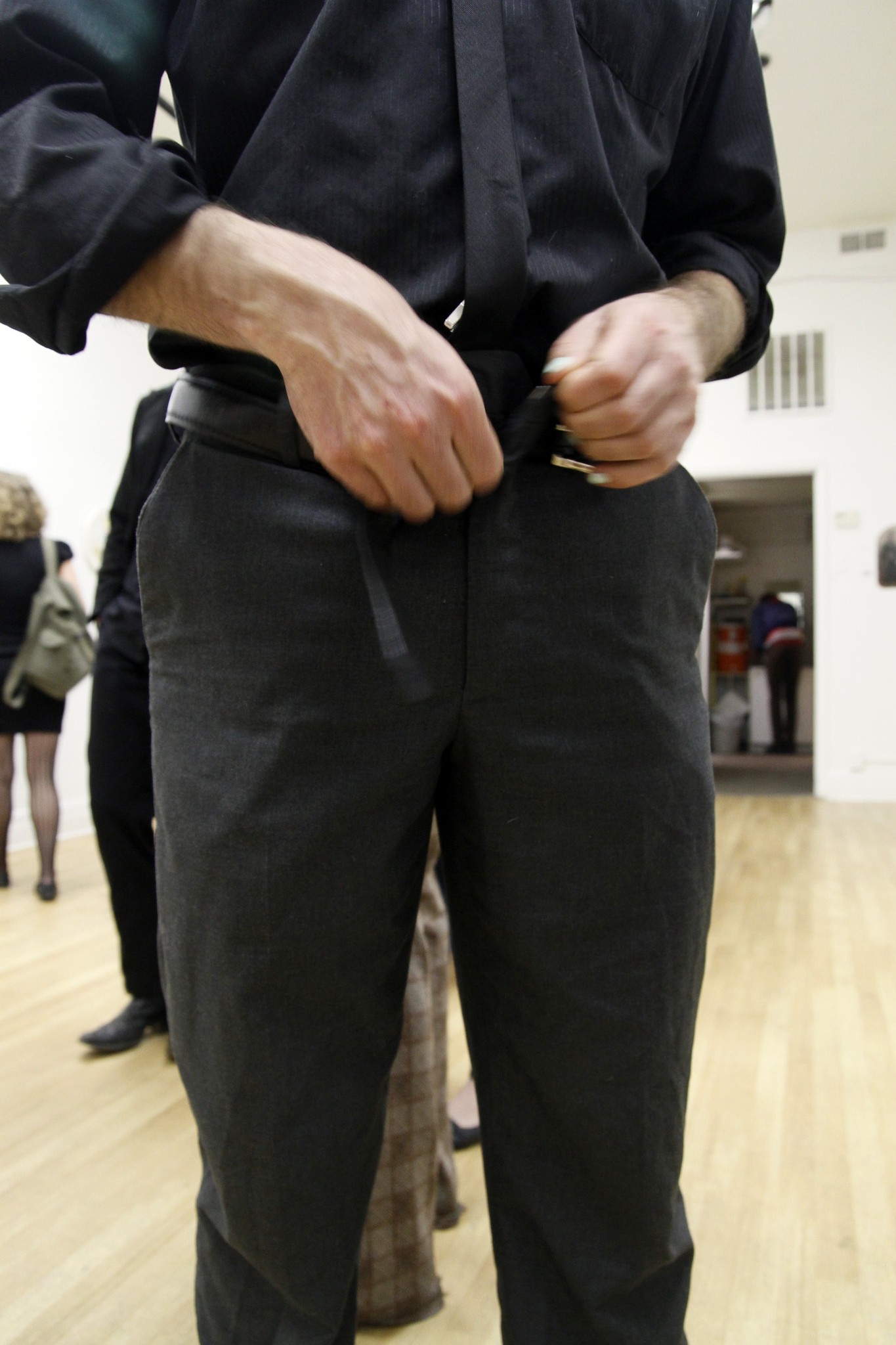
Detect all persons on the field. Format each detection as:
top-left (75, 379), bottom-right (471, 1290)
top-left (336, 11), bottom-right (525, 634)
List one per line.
top-left (753, 580), bottom-right (807, 757)
top-left (73, 381), bottom-right (187, 1054)
top-left (354, 815), bottom-right (482, 1330)
top-left (0, 472), bottom-right (80, 900)
top-left (0, 0), bottom-right (784, 1344)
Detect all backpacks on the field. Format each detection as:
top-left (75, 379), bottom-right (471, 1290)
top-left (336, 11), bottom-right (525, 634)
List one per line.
top-left (3, 539), bottom-right (99, 707)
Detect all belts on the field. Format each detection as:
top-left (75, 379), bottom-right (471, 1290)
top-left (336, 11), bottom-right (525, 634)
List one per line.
top-left (160, 371), bottom-right (612, 477)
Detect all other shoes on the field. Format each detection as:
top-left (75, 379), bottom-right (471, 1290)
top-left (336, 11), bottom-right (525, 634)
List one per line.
top-left (36, 881), bottom-right (56, 902)
top-left (0, 872), bottom-right (8, 887)
top-left (81, 994), bottom-right (166, 1050)
top-left (445, 1116), bottom-right (482, 1150)
